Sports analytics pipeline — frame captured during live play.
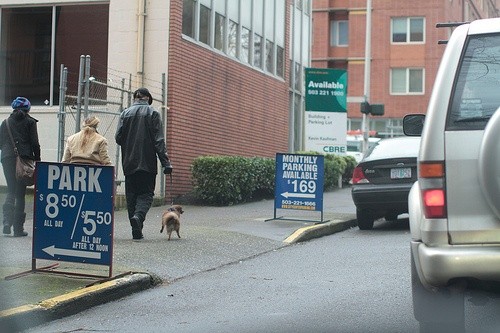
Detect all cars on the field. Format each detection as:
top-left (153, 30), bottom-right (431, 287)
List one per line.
top-left (350, 134), bottom-right (421, 230)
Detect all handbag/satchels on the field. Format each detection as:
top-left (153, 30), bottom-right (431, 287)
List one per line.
top-left (16, 156), bottom-right (36, 182)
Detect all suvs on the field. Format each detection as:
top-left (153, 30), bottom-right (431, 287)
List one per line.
top-left (400, 17), bottom-right (500, 332)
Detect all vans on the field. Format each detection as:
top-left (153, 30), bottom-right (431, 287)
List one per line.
top-left (346, 135), bottom-right (381, 162)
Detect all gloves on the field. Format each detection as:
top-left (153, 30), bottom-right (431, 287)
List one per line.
top-left (164, 166), bottom-right (173, 174)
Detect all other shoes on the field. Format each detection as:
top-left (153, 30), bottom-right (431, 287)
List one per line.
top-left (130, 216), bottom-right (142, 239)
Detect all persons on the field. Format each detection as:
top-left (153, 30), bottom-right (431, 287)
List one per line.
top-left (0, 96), bottom-right (41, 237)
top-left (114, 88), bottom-right (172, 239)
top-left (62, 116), bottom-right (112, 165)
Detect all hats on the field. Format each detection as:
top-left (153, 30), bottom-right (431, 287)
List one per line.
top-left (11, 97), bottom-right (31, 110)
top-left (134, 88), bottom-right (152, 105)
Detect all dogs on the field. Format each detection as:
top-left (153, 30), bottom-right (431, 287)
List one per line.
top-left (159, 205), bottom-right (185, 241)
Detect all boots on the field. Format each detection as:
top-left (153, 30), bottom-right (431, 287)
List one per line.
top-left (13, 211), bottom-right (29, 237)
top-left (3, 202), bottom-right (14, 235)
